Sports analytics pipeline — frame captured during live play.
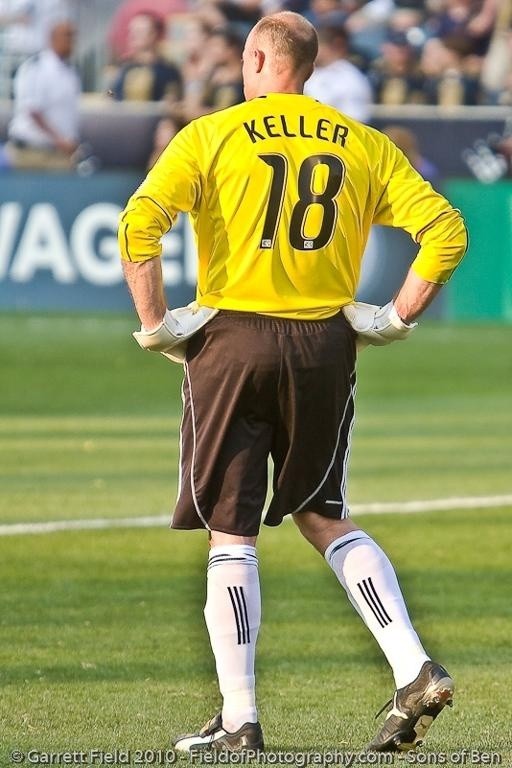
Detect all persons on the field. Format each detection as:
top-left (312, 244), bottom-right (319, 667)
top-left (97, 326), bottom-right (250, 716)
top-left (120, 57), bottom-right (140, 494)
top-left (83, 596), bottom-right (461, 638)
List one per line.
top-left (118, 10), bottom-right (469, 759)
top-left (5, 0), bottom-right (512, 175)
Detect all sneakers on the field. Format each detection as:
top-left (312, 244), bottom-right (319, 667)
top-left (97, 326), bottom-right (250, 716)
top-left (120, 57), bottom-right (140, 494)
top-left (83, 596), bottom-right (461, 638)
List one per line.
top-left (366, 660), bottom-right (456, 753)
top-left (170, 711), bottom-right (265, 757)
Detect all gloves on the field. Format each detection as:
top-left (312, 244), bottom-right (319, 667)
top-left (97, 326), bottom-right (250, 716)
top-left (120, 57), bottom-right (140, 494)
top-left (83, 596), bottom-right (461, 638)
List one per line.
top-left (131, 300), bottom-right (221, 364)
top-left (341, 299), bottom-right (418, 352)
